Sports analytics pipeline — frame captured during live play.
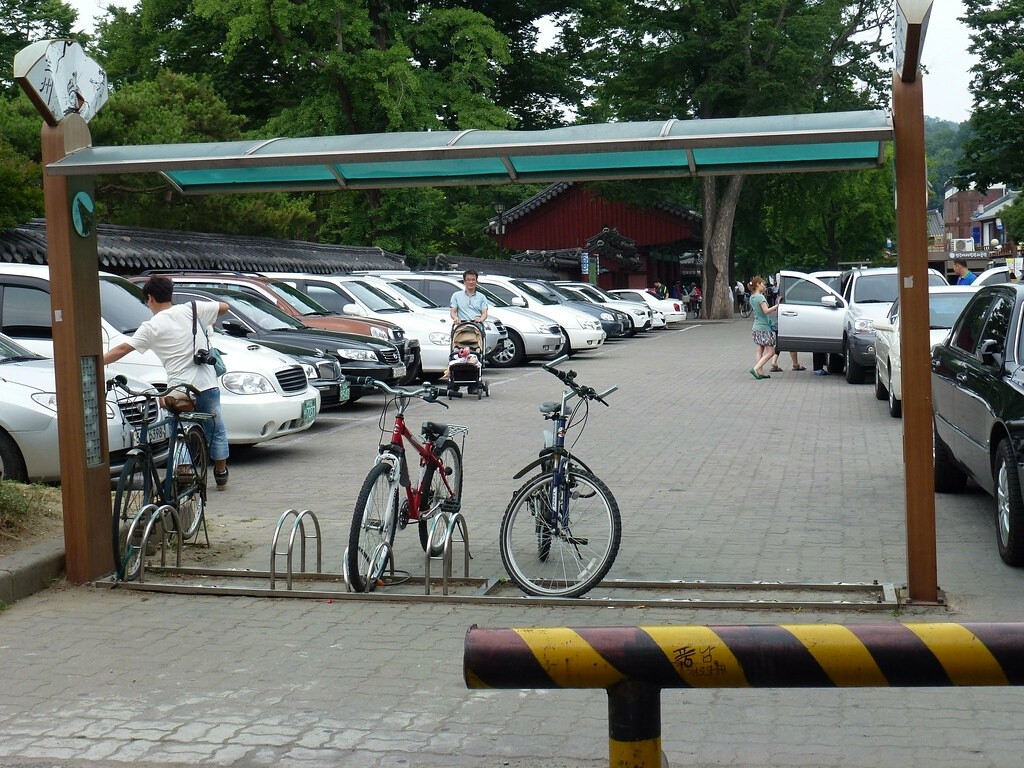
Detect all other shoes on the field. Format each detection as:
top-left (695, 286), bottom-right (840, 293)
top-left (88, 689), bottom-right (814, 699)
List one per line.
top-left (468, 387), bottom-right (478, 394)
top-left (758, 372), bottom-right (770, 378)
top-left (750, 368), bottom-right (762, 379)
top-left (814, 370), bottom-right (830, 375)
top-left (448, 385), bottom-right (458, 391)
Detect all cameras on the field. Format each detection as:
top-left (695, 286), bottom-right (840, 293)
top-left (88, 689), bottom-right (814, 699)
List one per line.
top-left (193, 350), bottom-right (218, 366)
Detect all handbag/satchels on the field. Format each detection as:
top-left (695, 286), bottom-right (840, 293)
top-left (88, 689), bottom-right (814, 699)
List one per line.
top-left (209, 347), bottom-right (227, 377)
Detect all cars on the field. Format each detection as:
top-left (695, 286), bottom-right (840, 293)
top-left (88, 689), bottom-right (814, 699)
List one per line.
top-left (0, 261), bottom-right (321, 448)
top-left (870, 286), bottom-right (987, 419)
top-left (0, 331), bottom-right (175, 485)
top-left (171, 287), bottom-right (423, 411)
top-left (219, 265), bottom-right (688, 381)
top-left (928, 284), bottom-right (1024, 568)
top-left (776, 265), bottom-right (1011, 386)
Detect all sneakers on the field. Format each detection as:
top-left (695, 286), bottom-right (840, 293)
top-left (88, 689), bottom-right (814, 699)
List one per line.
top-left (214, 466), bottom-right (228, 488)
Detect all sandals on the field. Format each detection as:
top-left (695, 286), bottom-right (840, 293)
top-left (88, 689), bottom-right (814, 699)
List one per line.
top-left (792, 364), bottom-right (806, 370)
top-left (769, 365), bottom-right (783, 372)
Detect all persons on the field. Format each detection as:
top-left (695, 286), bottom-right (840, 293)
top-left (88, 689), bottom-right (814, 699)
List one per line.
top-left (654, 279), bottom-right (669, 329)
top-left (813, 280), bottom-right (845, 375)
top-left (671, 281), bottom-right (701, 312)
top-left (104, 274), bottom-right (230, 491)
top-left (735, 280), bottom-right (745, 304)
top-left (765, 282), bottom-right (779, 307)
top-left (747, 276), bottom-right (782, 379)
top-left (770, 345), bottom-right (806, 372)
top-left (953, 258), bottom-right (977, 285)
top-left (447, 270), bottom-right (489, 394)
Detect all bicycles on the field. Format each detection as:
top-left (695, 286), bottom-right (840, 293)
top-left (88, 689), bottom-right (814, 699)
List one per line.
top-left (500, 354), bottom-right (622, 598)
top-left (344, 373), bottom-right (468, 593)
top-left (104, 374), bottom-right (218, 582)
top-left (740, 292), bottom-right (754, 318)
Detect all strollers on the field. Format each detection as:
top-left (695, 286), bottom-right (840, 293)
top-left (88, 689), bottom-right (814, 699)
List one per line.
top-left (446, 320), bottom-right (490, 399)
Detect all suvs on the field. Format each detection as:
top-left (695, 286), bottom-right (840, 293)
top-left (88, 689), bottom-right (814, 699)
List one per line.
top-left (125, 269), bottom-right (421, 375)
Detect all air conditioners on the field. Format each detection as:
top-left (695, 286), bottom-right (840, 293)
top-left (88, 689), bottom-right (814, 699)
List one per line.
top-left (951, 239), bottom-right (975, 252)
